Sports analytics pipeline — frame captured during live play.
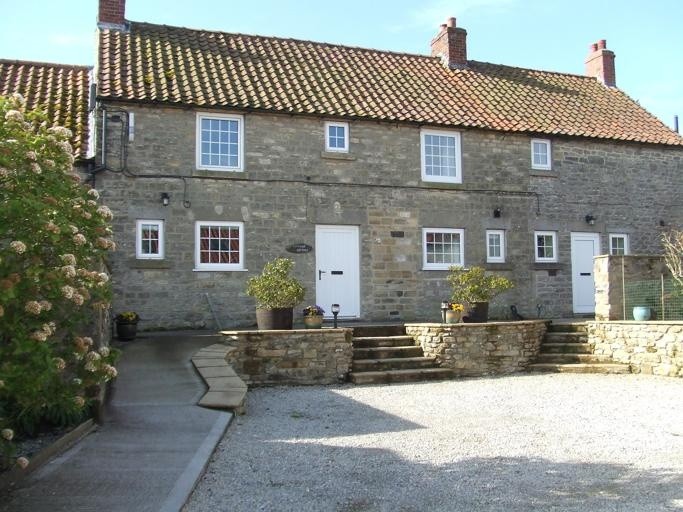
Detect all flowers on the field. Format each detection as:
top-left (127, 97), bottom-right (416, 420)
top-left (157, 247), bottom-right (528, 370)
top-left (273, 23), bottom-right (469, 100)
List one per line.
top-left (442, 302), bottom-right (463, 309)
top-left (302, 304), bottom-right (325, 315)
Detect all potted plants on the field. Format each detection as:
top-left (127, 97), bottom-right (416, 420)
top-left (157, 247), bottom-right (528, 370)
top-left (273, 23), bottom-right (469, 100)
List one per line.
top-left (114, 311), bottom-right (139, 340)
top-left (246, 258), bottom-right (306, 330)
top-left (447, 263), bottom-right (514, 322)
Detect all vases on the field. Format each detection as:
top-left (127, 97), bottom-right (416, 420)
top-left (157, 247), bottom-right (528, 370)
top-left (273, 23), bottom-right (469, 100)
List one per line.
top-left (304, 314), bottom-right (323, 328)
top-left (442, 309), bottom-right (462, 322)
top-left (633, 307), bottom-right (651, 321)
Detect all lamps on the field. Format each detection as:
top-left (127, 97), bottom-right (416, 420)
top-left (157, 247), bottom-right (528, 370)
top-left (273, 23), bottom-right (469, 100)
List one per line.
top-left (495, 208), bottom-right (502, 217)
top-left (586, 214), bottom-right (595, 226)
top-left (161, 192), bottom-right (169, 206)
